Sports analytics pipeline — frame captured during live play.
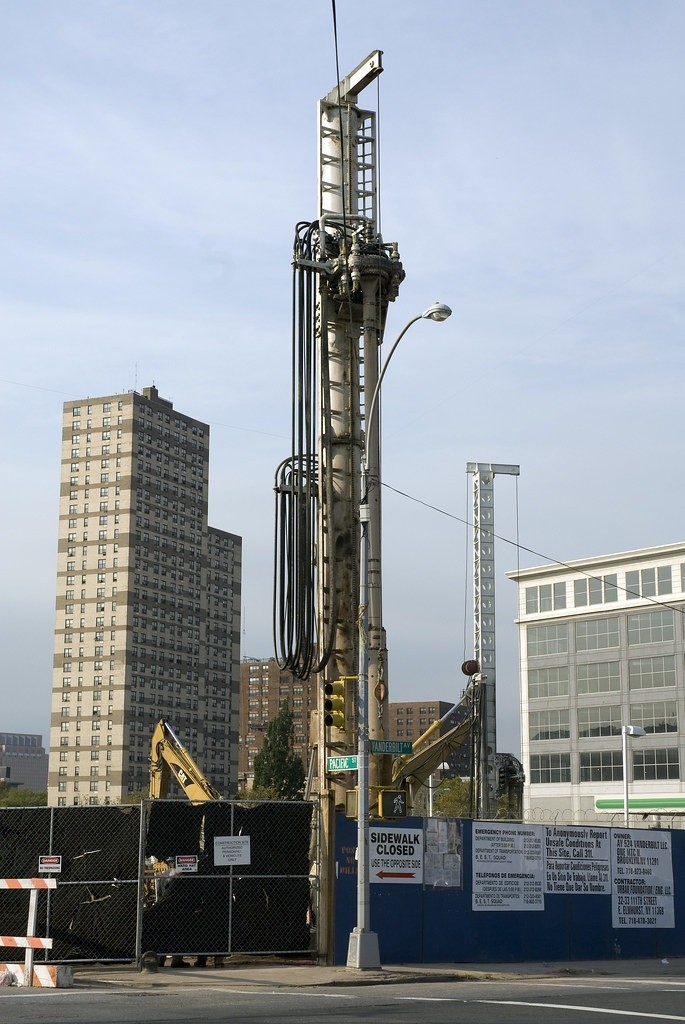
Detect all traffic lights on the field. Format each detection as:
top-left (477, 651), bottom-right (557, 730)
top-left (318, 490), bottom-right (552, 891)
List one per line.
top-left (323, 680), bottom-right (347, 730)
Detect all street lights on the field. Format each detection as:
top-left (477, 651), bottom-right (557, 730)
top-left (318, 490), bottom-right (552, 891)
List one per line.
top-left (621, 725), bottom-right (646, 828)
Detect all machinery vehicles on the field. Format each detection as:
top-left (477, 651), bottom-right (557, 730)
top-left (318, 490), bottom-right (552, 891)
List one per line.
top-left (344, 673), bottom-right (488, 821)
top-left (148, 717), bottom-right (223, 805)
